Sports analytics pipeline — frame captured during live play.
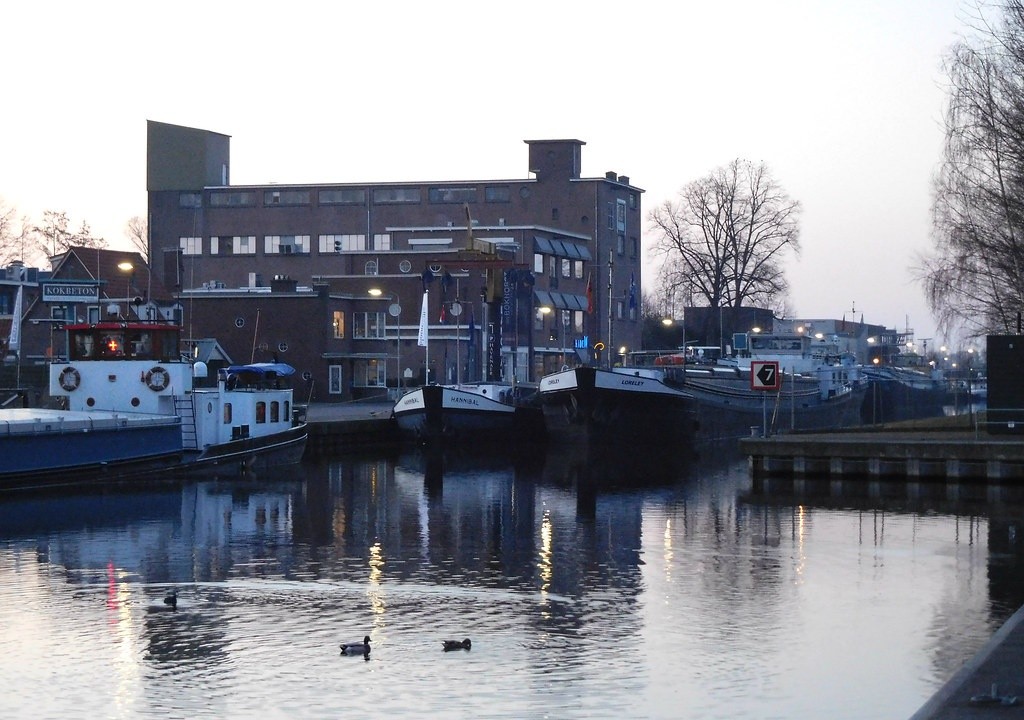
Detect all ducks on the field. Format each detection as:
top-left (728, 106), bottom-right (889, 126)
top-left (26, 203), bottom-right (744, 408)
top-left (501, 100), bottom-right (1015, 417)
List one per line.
top-left (164, 592), bottom-right (179, 603)
top-left (340, 636), bottom-right (372, 650)
top-left (443, 638), bottom-right (471, 652)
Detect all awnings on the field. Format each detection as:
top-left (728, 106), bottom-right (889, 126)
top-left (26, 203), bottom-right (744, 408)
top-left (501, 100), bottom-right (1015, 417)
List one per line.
top-left (535, 237), bottom-right (592, 260)
top-left (535, 290), bottom-right (588, 310)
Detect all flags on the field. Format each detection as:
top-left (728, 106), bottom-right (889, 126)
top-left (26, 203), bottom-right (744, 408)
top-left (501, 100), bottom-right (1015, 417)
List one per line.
top-left (439, 305), bottom-right (446, 324)
top-left (585, 278), bottom-right (593, 313)
top-left (629, 270), bottom-right (636, 308)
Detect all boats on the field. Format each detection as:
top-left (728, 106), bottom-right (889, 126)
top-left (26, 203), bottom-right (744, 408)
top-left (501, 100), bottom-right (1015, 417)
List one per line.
top-left (391, 301), bottom-right (989, 464)
top-left (1, 212), bottom-right (317, 493)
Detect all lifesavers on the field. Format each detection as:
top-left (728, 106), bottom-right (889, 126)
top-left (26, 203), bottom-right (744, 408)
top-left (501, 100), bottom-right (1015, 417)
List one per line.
top-left (59, 366), bottom-right (81, 391)
top-left (146, 366), bottom-right (170, 392)
